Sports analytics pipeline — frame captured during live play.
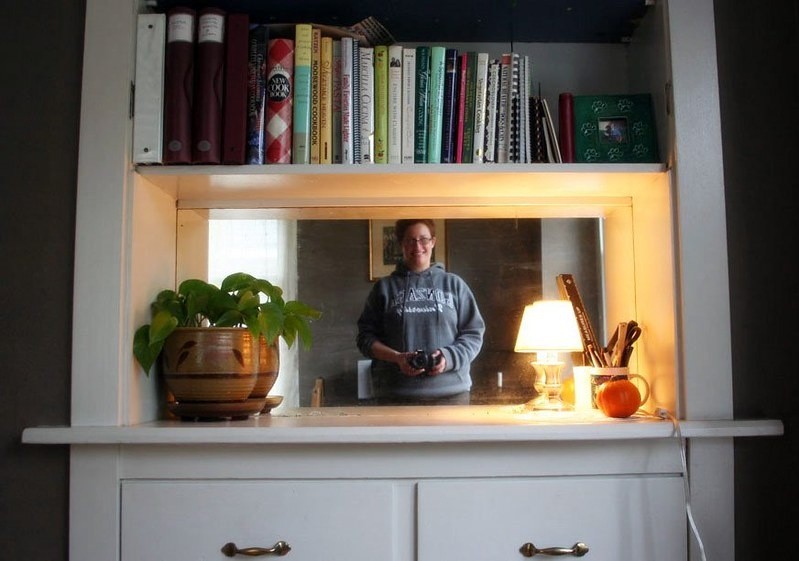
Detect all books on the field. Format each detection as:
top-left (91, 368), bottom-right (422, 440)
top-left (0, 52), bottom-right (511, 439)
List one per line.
top-left (132, 11), bottom-right (574, 164)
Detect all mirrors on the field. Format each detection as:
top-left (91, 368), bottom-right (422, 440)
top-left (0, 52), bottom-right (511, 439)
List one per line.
top-left (178, 197), bottom-right (634, 405)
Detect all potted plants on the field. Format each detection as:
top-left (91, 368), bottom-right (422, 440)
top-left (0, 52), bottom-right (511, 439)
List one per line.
top-left (249, 295), bottom-right (320, 413)
top-left (136, 271), bottom-right (263, 417)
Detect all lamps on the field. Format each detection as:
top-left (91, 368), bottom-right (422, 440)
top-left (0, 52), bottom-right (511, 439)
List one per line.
top-left (514, 304), bottom-right (546, 408)
top-left (518, 301), bottom-right (583, 410)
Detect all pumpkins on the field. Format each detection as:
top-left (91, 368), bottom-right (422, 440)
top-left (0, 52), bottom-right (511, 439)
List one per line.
top-left (596, 376), bottom-right (641, 418)
top-left (561, 372), bottom-right (575, 404)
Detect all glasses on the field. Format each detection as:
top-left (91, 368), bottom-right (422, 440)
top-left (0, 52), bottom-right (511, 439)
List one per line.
top-left (400, 237), bottom-right (433, 246)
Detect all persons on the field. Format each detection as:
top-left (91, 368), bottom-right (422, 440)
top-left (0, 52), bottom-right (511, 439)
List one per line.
top-left (356, 217), bottom-right (487, 405)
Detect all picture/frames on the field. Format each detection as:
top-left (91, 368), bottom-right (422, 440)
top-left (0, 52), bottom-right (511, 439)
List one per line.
top-left (369, 219), bottom-right (449, 280)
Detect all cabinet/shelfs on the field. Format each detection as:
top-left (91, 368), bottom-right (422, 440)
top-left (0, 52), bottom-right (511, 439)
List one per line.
top-left (22, 0), bottom-right (784, 561)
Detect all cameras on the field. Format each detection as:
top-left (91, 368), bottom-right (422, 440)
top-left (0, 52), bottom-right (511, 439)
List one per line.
top-left (409, 350), bottom-right (440, 374)
